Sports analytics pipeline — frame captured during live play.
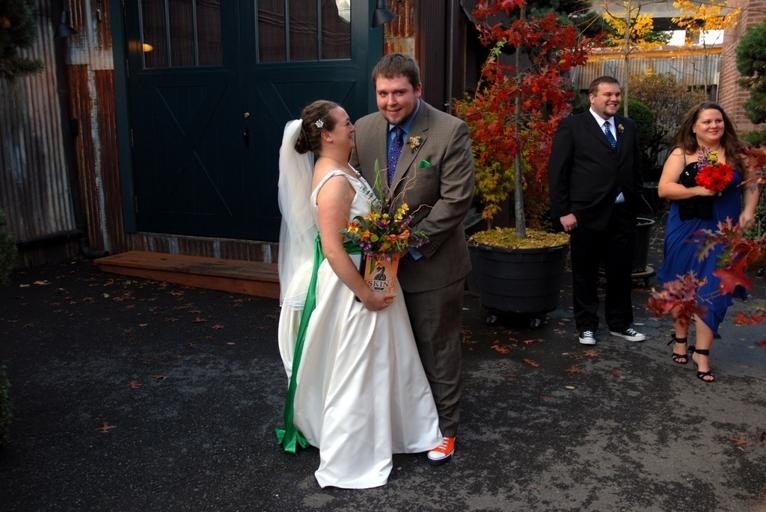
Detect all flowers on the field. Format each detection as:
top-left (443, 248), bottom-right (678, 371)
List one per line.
top-left (405, 134), bottom-right (422, 152)
top-left (694, 144), bottom-right (735, 196)
top-left (333, 152), bottom-right (441, 259)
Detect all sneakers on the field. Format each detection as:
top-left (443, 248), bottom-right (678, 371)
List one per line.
top-left (579, 331), bottom-right (596, 344)
top-left (427, 436), bottom-right (456, 465)
top-left (609, 327), bottom-right (645, 341)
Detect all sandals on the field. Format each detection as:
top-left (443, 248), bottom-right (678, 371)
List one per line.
top-left (667, 333), bottom-right (688, 364)
top-left (688, 345), bottom-right (715, 382)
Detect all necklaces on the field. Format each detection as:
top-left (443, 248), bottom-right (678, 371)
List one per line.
top-left (318, 153), bottom-right (362, 180)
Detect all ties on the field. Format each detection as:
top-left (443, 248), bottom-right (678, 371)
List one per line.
top-left (388, 128), bottom-right (404, 188)
top-left (604, 121), bottom-right (616, 151)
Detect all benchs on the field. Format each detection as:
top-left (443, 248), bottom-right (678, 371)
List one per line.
top-left (91, 247), bottom-right (282, 303)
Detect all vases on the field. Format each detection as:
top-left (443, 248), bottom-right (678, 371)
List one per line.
top-left (624, 210), bottom-right (658, 279)
top-left (353, 239), bottom-right (401, 306)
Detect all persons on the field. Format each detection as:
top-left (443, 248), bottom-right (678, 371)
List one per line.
top-left (348, 53), bottom-right (473, 461)
top-left (276, 101), bottom-right (442, 490)
top-left (550, 75), bottom-right (649, 344)
top-left (658, 101), bottom-right (760, 385)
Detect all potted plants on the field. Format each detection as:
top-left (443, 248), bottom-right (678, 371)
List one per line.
top-left (450, 1), bottom-right (594, 326)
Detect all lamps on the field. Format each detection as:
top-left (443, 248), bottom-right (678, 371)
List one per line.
top-left (369, 0), bottom-right (397, 30)
